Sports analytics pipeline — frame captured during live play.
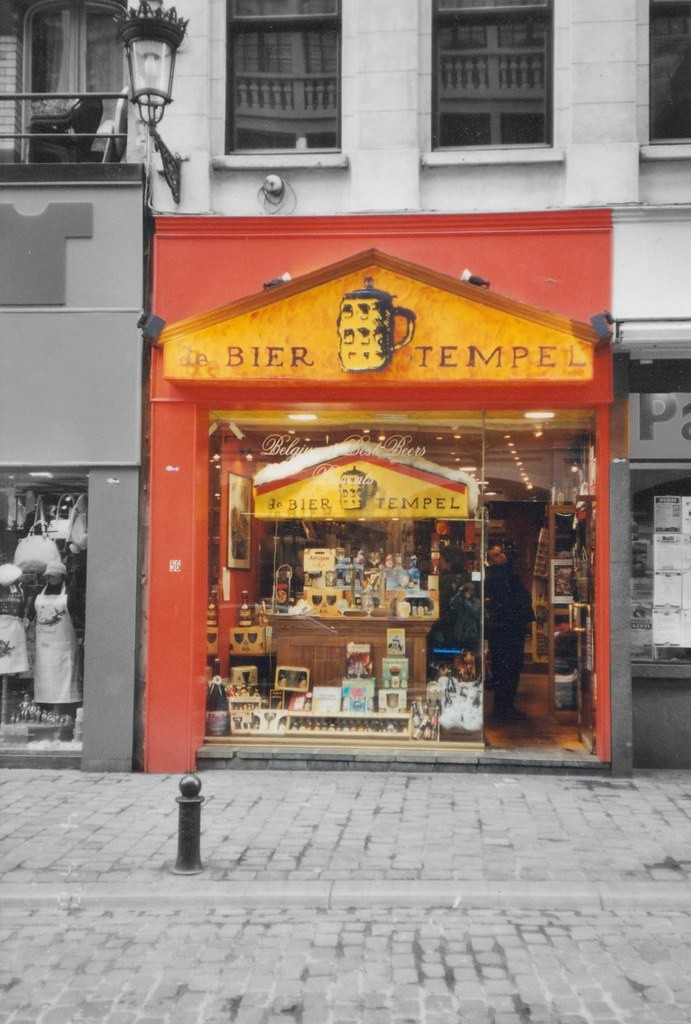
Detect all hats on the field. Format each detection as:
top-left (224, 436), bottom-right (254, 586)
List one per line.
top-left (43, 561), bottom-right (67, 576)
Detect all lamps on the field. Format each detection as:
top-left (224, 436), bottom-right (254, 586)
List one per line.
top-left (107, 0), bottom-right (181, 204)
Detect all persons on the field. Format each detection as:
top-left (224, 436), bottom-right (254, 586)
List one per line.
top-left (434, 543), bottom-right (471, 650)
top-left (478, 545), bottom-right (537, 722)
top-left (450, 583), bottom-right (482, 650)
top-left (0, 580), bottom-right (29, 674)
top-left (26, 561), bottom-right (82, 704)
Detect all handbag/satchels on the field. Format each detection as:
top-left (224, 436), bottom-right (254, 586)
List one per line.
top-left (13, 492), bottom-right (87, 573)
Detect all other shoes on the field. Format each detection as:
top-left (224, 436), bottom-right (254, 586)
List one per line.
top-left (492, 709), bottom-right (527, 724)
